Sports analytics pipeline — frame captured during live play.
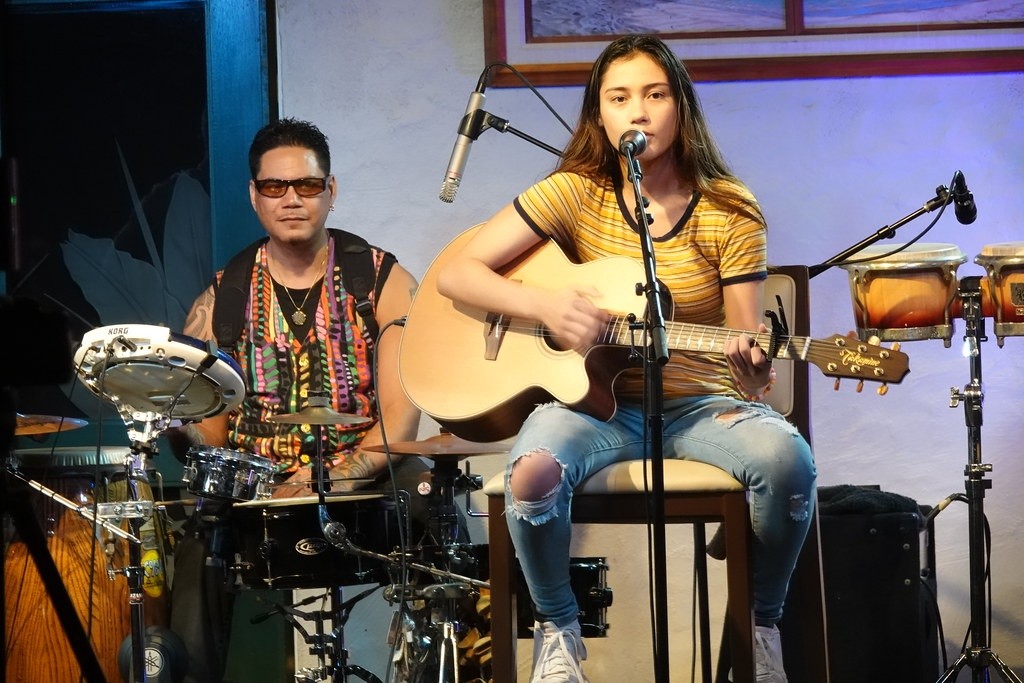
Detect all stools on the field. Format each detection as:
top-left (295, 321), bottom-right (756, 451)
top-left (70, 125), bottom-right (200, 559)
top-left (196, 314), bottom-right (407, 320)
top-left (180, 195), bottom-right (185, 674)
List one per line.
top-left (239, 521), bottom-right (400, 683)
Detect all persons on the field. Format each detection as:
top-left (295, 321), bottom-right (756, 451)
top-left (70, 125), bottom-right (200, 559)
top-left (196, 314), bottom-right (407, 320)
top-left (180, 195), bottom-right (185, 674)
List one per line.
top-left (437, 35), bottom-right (817, 683)
top-left (172, 114), bottom-right (472, 683)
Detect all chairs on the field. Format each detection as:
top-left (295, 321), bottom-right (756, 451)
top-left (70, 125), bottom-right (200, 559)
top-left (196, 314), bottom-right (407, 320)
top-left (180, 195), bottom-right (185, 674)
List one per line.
top-left (483, 259), bottom-right (811, 683)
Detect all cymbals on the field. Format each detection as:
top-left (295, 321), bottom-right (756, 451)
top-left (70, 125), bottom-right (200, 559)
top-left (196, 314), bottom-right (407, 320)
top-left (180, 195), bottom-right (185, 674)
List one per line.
top-left (359, 434), bottom-right (515, 462)
top-left (15, 411), bottom-right (89, 436)
top-left (265, 405), bottom-right (373, 426)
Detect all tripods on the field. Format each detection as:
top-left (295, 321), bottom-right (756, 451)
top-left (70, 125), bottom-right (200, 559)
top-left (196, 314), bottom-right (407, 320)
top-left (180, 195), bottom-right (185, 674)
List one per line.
top-left (904, 293), bottom-right (1024, 683)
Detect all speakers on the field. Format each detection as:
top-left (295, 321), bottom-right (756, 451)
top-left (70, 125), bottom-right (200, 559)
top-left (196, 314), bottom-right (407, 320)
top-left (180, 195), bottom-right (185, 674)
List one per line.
top-left (777, 512), bottom-right (939, 683)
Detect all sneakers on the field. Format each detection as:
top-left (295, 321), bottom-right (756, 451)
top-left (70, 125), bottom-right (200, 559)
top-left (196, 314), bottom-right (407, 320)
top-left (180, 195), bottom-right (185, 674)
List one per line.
top-left (754, 624), bottom-right (788, 683)
top-left (528, 618), bottom-right (590, 683)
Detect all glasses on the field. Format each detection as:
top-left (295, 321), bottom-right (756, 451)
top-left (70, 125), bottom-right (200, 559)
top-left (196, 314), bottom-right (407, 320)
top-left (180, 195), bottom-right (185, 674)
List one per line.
top-left (253, 174), bottom-right (329, 198)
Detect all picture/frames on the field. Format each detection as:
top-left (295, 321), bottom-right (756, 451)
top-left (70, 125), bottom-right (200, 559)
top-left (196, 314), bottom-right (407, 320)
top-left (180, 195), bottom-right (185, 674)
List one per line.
top-left (481, 0), bottom-right (1024, 91)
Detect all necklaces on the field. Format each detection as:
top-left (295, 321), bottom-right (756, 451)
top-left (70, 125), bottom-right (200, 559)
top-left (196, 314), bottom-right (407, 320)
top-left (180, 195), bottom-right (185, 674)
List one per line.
top-left (270, 239), bottom-right (328, 326)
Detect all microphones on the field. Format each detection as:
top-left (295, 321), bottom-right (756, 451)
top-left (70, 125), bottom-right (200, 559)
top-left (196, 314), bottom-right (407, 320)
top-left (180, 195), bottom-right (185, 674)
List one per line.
top-left (437, 66), bottom-right (490, 203)
top-left (618, 128), bottom-right (646, 157)
top-left (954, 170), bottom-right (977, 225)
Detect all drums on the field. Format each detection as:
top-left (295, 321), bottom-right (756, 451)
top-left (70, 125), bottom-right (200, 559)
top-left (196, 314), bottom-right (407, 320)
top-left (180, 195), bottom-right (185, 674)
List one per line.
top-left (0, 444), bottom-right (170, 683)
top-left (839, 241), bottom-right (1024, 337)
top-left (180, 442), bottom-right (278, 506)
top-left (415, 554), bottom-right (613, 640)
top-left (236, 488), bottom-right (411, 592)
top-left (70, 321), bottom-right (247, 426)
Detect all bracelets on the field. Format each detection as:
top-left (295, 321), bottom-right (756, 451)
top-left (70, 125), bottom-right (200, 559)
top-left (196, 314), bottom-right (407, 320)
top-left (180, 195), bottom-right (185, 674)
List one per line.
top-left (737, 366), bottom-right (776, 401)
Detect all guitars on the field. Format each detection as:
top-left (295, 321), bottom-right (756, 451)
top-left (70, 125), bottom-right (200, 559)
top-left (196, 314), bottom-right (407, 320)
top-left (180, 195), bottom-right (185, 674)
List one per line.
top-left (395, 219), bottom-right (912, 444)
